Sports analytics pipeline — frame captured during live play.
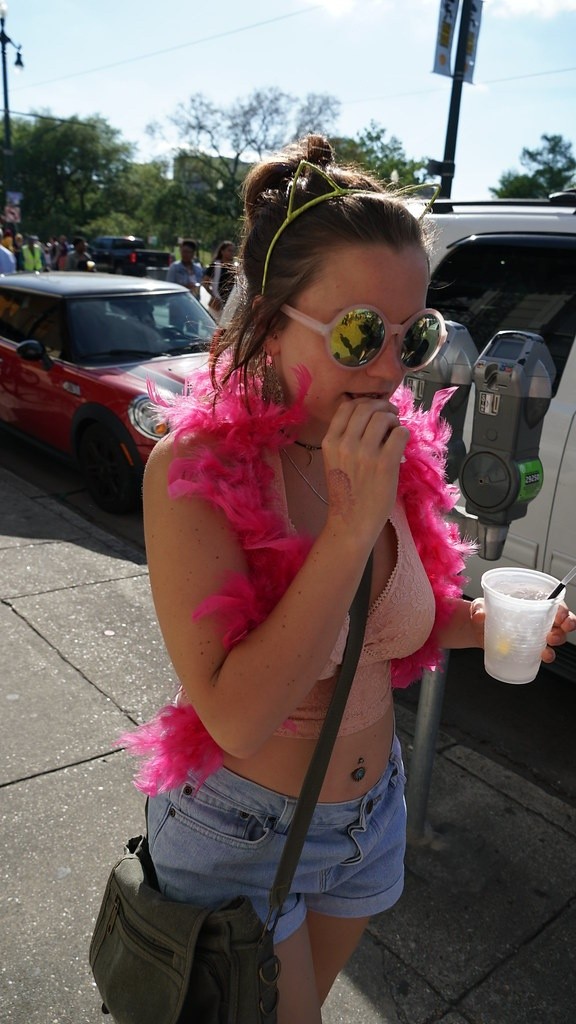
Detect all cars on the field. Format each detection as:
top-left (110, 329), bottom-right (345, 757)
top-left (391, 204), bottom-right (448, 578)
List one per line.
top-left (0, 270), bottom-right (220, 516)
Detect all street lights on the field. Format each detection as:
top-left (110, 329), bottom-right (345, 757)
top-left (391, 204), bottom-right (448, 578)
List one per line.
top-left (0, 3), bottom-right (25, 149)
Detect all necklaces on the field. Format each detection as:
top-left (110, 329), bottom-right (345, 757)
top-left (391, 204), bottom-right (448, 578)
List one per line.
top-left (280, 429), bottom-right (325, 452)
top-left (283, 448), bottom-right (331, 507)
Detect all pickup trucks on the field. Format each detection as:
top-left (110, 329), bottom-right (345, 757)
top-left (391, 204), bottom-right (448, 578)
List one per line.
top-left (89, 236), bottom-right (172, 278)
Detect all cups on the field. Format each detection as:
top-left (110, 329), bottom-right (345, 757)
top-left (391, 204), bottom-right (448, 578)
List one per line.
top-left (480, 566), bottom-right (566, 684)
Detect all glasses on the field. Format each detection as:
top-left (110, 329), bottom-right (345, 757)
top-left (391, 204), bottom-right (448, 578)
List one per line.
top-left (268, 297), bottom-right (448, 372)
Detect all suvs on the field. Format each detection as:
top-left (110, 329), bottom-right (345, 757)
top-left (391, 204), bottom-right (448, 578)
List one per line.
top-left (407, 191), bottom-right (575, 650)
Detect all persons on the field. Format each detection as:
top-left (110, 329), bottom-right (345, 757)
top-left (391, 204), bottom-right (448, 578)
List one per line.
top-left (44, 234), bottom-right (71, 271)
top-left (162, 240), bottom-right (206, 335)
top-left (118, 132), bottom-right (576, 1024)
top-left (0, 225), bottom-right (47, 272)
top-left (199, 239), bottom-right (239, 321)
top-left (62, 237), bottom-right (98, 272)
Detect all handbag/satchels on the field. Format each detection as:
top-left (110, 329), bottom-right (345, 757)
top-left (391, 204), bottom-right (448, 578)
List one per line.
top-left (88, 835), bottom-right (281, 1024)
